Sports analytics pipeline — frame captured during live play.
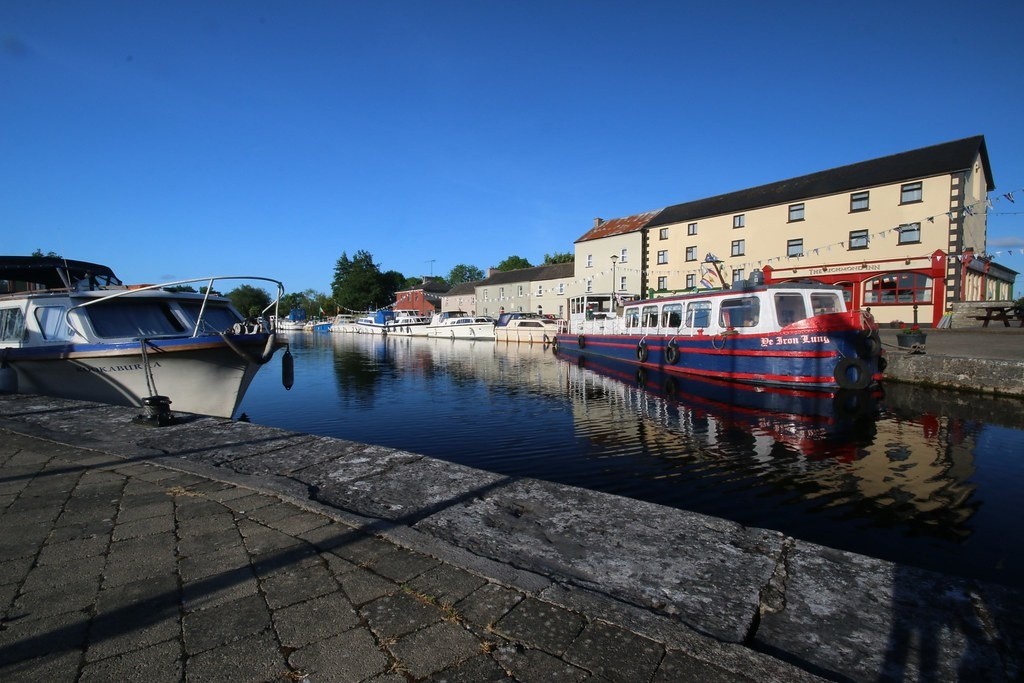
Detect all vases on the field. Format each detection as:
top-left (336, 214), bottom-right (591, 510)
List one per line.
top-left (896, 333), bottom-right (926, 346)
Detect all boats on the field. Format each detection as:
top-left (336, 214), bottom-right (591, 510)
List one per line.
top-left (553, 344), bottom-right (879, 460)
top-left (259, 309), bottom-right (566, 343)
top-left (552, 252), bottom-right (888, 390)
top-left (0, 256), bottom-right (294, 420)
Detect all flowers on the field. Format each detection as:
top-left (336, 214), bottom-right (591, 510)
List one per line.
top-left (899, 321), bottom-right (923, 334)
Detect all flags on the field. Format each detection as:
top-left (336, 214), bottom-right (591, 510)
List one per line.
top-left (699, 263), bottom-right (726, 289)
top-left (320, 307), bottom-right (326, 315)
top-left (337, 306), bottom-right (339, 313)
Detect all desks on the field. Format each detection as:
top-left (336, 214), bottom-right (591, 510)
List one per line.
top-left (976, 306), bottom-right (1015, 328)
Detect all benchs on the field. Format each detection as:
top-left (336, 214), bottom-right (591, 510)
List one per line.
top-left (966, 315), bottom-right (1016, 321)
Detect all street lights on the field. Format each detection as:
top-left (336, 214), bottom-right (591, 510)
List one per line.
top-left (610, 254), bottom-right (620, 313)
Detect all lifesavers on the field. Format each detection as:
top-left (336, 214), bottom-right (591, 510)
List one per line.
top-left (664, 344), bottom-right (679, 365)
top-left (552, 337), bottom-right (557, 346)
top-left (834, 356), bottom-right (869, 390)
top-left (851, 329), bottom-right (882, 360)
top-left (578, 335), bottom-right (586, 349)
top-left (635, 344), bottom-right (647, 363)
